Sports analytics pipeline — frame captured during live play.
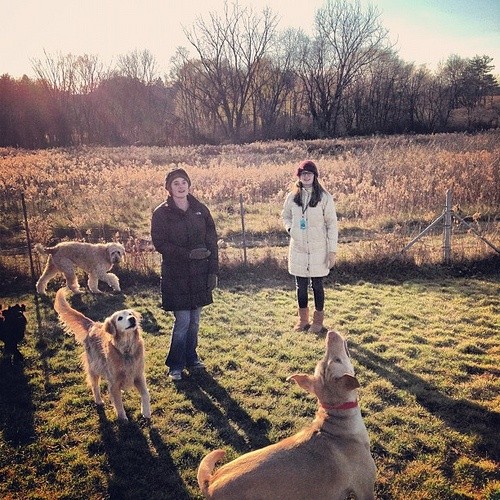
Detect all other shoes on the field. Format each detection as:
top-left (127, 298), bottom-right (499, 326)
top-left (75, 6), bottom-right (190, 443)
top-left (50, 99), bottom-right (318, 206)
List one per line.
top-left (169, 370), bottom-right (181, 379)
top-left (184, 362), bottom-right (205, 369)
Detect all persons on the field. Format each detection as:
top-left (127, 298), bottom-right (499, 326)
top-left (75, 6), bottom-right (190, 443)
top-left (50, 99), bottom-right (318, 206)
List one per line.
top-left (151, 168), bottom-right (219, 379)
top-left (280, 161), bottom-right (338, 333)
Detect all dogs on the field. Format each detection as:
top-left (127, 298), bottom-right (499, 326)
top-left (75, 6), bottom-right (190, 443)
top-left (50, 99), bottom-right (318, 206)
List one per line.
top-left (31, 239), bottom-right (127, 297)
top-left (52, 285), bottom-right (152, 427)
top-left (0, 299), bottom-right (29, 357)
top-left (196, 330), bottom-right (382, 500)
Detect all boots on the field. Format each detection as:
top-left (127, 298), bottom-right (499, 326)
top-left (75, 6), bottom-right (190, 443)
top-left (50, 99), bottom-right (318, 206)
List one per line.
top-left (308, 310), bottom-right (324, 333)
top-left (294, 307), bottom-right (309, 330)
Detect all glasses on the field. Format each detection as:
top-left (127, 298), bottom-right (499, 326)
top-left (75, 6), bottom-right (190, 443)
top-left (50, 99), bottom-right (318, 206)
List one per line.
top-left (301, 172), bottom-right (314, 177)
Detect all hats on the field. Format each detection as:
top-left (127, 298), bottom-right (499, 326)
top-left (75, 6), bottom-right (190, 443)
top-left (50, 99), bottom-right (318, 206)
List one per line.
top-left (296, 161), bottom-right (318, 177)
top-left (164, 168), bottom-right (191, 190)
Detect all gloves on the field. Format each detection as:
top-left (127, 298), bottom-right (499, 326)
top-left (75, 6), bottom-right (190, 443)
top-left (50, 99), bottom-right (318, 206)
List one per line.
top-left (191, 248), bottom-right (208, 260)
top-left (207, 273), bottom-right (217, 291)
